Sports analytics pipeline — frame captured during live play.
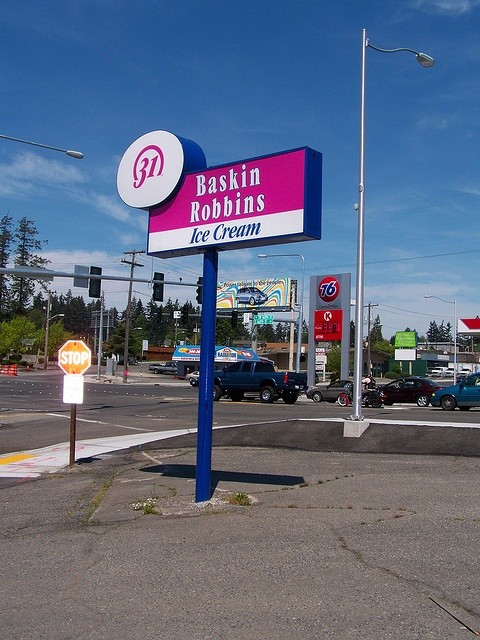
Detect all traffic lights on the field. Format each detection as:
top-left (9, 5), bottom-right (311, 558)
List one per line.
top-left (181, 305), bottom-right (188, 325)
top-left (231, 310), bottom-right (238, 328)
top-left (196, 277), bottom-right (203, 304)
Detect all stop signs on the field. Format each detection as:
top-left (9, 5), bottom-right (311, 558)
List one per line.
top-left (58, 340), bottom-right (91, 375)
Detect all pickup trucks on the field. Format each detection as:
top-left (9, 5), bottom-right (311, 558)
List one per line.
top-left (213, 361), bottom-right (309, 403)
top-left (149, 362), bottom-right (178, 374)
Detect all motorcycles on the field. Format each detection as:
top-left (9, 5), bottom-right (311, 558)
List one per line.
top-left (338, 381), bottom-right (384, 408)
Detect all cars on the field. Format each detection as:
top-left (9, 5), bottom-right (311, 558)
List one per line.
top-left (431, 372), bottom-right (480, 411)
top-left (186, 371), bottom-right (200, 387)
top-left (382, 378), bottom-right (442, 406)
top-left (307, 380), bottom-right (353, 403)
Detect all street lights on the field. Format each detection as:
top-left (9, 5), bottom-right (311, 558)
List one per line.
top-left (258, 253), bottom-right (305, 373)
top-left (424, 295), bottom-right (457, 386)
top-left (350, 29), bottom-right (434, 421)
top-left (44, 314), bottom-right (65, 369)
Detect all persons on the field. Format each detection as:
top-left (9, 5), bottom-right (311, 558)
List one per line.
top-left (362, 373), bottom-right (376, 398)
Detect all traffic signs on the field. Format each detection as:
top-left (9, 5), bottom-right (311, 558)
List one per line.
top-left (254, 315), bottom-right (273, 324)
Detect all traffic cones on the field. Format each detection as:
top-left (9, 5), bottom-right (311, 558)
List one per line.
top-left (15, 364), bottom-right (18, 376)
top-left (10, 364), bottom-right (15, 376)
top-left (0, 365), bottom-right (2, 374)
top-left (4, 365), bottom-right (9, 374)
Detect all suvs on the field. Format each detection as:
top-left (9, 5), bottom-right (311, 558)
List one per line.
top-left (235, 287), bottom-right (268, 305)
